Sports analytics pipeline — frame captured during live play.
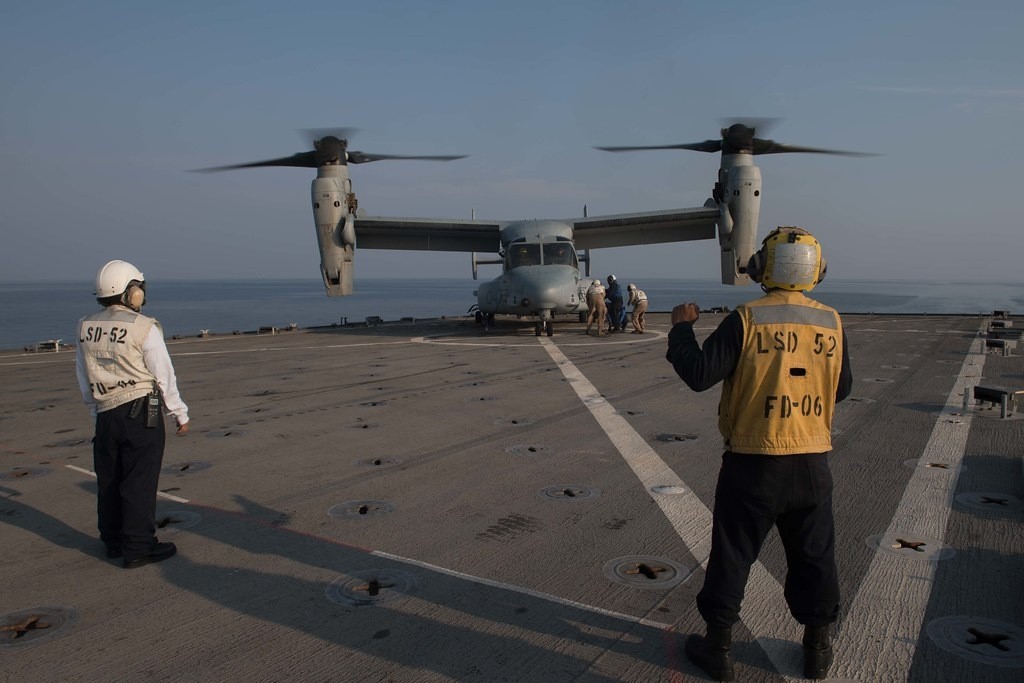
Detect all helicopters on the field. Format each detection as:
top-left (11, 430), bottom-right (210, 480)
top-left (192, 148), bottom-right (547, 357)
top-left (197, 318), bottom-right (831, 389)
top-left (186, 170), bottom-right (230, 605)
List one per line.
top-left (183, 114), bottom-right (889, 341)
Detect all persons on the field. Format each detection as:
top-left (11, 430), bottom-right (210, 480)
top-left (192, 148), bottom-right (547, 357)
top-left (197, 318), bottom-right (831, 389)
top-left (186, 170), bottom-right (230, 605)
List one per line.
top-left (664, 226), bottom-right (854, 683)
top-left (75, 260), bottom-right (190, 567)
top-left (625, 283), bottom-right (648, 334)
top-left (584, 275), bottom-right (628, 337)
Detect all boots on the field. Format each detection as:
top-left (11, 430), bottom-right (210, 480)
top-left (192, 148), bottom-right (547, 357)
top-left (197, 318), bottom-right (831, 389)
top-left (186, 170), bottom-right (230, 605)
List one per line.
top-left (804, 626), bottom-right (834, 679)
top-left (685, 626), bottom-right (734, 681)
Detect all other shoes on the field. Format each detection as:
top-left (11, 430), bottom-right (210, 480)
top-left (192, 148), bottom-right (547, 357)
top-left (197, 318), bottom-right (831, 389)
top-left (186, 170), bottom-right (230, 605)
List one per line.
top-left (586, 327), bottom-right (591, 334)
top-left (631, 331), bottom-right (639, 334)
top-left (640, 331), bottom-right (645, 334)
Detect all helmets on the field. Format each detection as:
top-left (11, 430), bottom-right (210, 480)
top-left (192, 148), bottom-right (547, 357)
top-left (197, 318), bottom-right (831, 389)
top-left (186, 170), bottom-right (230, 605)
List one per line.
top-left (761, 226), bottom-right (822, 292)
top-left (593, 280), bottom-right (600, 285)
top-left (628, 283), bottom-right (636, 290)
top-left (607, 275), bottom-right (617, 285)
top-left (95, 260), bottom-right (145, 298)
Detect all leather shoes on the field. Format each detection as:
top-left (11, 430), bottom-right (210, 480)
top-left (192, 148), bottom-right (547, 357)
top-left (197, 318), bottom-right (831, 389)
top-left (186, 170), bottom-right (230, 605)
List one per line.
top-left (125, 542), bottom-right (175, 568)
top-left (107, 544), bottom-right (127, 559)
